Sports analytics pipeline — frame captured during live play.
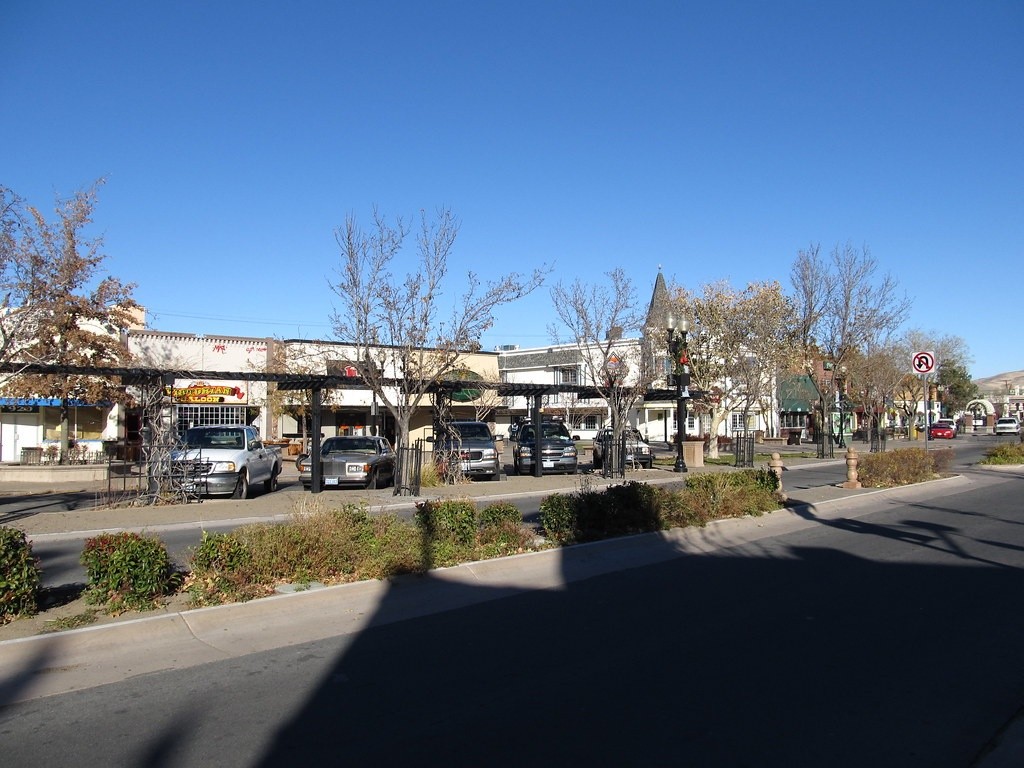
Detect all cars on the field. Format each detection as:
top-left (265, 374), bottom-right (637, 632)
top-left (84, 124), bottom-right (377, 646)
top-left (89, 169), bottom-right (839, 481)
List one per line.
top-left (928, 382), bottom-right (944, 441)
top-left (931, 418), bottom-right (957, 440)
top-left (426, 422), bottom-right (504, 481)
top-left (509, 423), bottom-right (580, 476)
top-left (298, 436), bottom-right (397, 490)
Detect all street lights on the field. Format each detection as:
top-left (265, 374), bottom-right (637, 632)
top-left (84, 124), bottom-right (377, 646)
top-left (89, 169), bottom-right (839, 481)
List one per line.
top-left (666, 317), bottom-right (690, 473)
top-left (362, 349), bottom-right (386, 436)
top-left (834, 365), bottom-right (847, 448)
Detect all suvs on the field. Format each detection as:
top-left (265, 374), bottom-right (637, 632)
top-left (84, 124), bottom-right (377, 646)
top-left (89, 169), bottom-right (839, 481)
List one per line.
top-left (593, 426), bottom-right (651, 469)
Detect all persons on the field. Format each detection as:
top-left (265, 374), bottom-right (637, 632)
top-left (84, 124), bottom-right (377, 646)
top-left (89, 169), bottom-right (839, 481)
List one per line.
top-left (511, 423), bottom-right (518, 436)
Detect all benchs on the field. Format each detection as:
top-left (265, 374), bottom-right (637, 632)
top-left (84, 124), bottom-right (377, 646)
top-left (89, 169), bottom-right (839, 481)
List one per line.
top-left (762, 437), bottom-right (787, 445)
top-left (885, 427), bottom-right (907, 440)
top-left (582, 446), bottom-right (593, 454)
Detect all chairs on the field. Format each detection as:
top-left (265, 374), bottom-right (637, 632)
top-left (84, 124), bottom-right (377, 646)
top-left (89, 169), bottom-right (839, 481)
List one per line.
top-left (525, 431), bottom-right (534, 439)
top-left (192, 437), bottom-right (215, 444)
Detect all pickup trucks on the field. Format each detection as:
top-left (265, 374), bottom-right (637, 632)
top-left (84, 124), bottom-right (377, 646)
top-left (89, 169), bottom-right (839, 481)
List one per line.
top-left (997, 417), bottom-right (1021, 435)
top-left (160, 425), bottom-right (282, 499)
